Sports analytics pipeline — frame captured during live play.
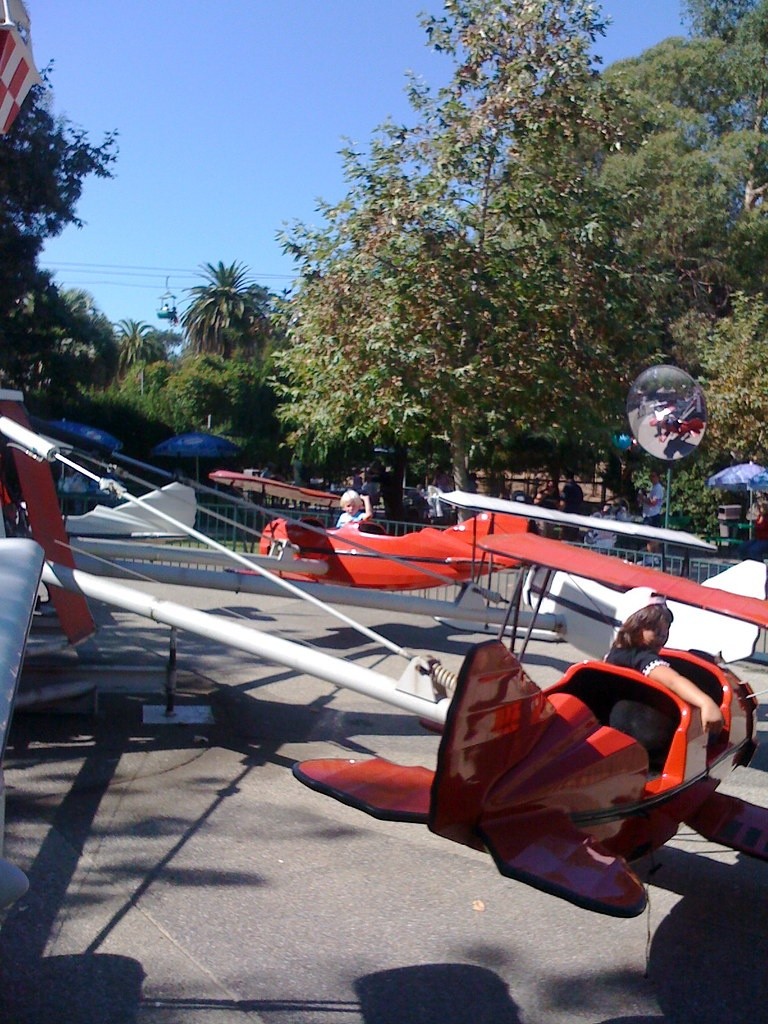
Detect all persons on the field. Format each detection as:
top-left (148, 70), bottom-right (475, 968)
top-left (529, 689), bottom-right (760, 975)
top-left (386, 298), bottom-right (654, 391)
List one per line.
top-left (414, 467), bottom-right (480, 526)
top-left (638, 471), bottom-right (664, 554)
top-left (753, 497), bottom-right (768, 539)
top-left (533, 470), bottom-right (583, 541)
top-left (336, 490), bottom-right (373, 528)
top-left (602, 593), bottom-right (726, 734)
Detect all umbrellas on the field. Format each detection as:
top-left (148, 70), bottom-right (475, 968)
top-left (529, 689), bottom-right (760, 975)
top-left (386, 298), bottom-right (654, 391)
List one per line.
top-left (51, 419), bottom-right (122, 476)
top-left (152, 430), bottom-right (240, 482)
top-left (706, 461), bottom-right (768, 539)
top-left (748, 468), bottom-right (768, 493)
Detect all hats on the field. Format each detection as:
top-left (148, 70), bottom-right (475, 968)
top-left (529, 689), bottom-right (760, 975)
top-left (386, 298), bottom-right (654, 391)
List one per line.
top-left (614, 587), bottom-right (667, 630)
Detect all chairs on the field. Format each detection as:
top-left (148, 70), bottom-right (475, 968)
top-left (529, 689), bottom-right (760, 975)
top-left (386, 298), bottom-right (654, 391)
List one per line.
top-left (298, 517), bottom-right (326, 531)
top-left (359, 522), bottom-right (385, 535)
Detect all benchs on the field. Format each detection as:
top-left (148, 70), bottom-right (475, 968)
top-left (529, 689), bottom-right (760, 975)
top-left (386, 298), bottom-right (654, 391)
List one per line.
top-left (659, 649), bottom-right (732, 760)
top-left (542, 661), bottom-right (690, 796)
